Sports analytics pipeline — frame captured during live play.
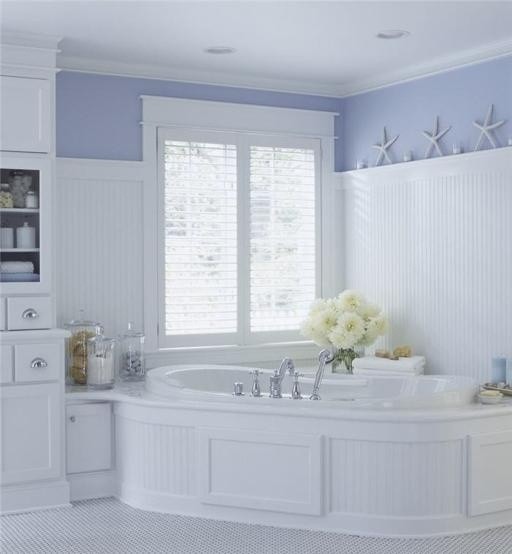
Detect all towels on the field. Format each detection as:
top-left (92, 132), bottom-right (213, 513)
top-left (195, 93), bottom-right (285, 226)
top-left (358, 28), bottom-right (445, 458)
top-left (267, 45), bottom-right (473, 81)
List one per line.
top-left (0, 273), bottom-right (40, 282)
top-left (0, 261), bottom-right (34, 273)
top-left (351, 354), bottom-right (426, 373)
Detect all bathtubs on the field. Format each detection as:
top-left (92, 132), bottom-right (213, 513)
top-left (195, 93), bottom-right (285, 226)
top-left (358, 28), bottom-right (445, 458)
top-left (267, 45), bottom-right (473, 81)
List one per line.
top-left (144, 362), bottom-right (512, 423)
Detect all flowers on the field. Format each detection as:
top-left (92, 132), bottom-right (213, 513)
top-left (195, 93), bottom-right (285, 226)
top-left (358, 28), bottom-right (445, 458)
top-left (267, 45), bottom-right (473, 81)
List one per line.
top-left (297, 286), bottom-right (388, 349)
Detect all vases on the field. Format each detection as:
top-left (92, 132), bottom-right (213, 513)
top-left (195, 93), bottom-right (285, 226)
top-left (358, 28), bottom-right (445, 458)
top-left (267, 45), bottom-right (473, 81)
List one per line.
top-left (330, 343), bottom-right (357, 375)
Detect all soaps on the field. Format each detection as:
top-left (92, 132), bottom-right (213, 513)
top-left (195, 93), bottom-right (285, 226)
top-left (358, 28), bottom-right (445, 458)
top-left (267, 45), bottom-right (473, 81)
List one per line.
top-left (480, 391), bottom-right (497, 396)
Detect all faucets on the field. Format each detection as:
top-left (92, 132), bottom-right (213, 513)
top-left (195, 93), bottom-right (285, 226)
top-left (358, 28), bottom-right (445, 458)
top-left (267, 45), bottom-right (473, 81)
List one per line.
top-left (268, 356), bottom-right (297, 397)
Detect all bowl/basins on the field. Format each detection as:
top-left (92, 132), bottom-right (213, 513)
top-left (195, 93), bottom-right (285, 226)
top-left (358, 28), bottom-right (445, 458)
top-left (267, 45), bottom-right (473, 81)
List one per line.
top-left (477, 391), bottom-right (503, 405)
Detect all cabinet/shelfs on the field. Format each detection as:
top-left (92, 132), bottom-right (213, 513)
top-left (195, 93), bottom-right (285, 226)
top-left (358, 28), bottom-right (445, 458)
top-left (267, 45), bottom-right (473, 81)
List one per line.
top-left (0, 329), bottom-right (71, 516)
top-left (65, 401), bottom-right (113, 475)
top-left (0, 29), bottom-right (58, 330)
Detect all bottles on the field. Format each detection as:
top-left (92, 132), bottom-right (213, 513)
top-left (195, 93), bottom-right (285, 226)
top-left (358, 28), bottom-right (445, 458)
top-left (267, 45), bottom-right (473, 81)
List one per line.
top-left (119, 319), bottom-right (145, 381)
top-left (8, 169), bottom-right (32, 206)
top-left (66, 306), bottom-right (105, 388)
top-left (24, 190), bottom-right (39, 209)
top-left (1, 181), bottom-right (14, 208)
top-left (88, 322), bottom-right (116, 391)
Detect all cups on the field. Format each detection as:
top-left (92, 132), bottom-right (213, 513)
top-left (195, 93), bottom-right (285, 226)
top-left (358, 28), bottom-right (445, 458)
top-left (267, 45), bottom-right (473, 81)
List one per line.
top-left (508, 134), bottom-right (512, 145)
top-left (357, 155), bottom-right (370, 169)
top-left (0, 222), bottom-right (14, 250)
top-left (17, 222), bottom-right (35, 250)
top-left (451, 140), bottom-right (461, 153)
top-left (402, 149), bottom-right (412, 161)
